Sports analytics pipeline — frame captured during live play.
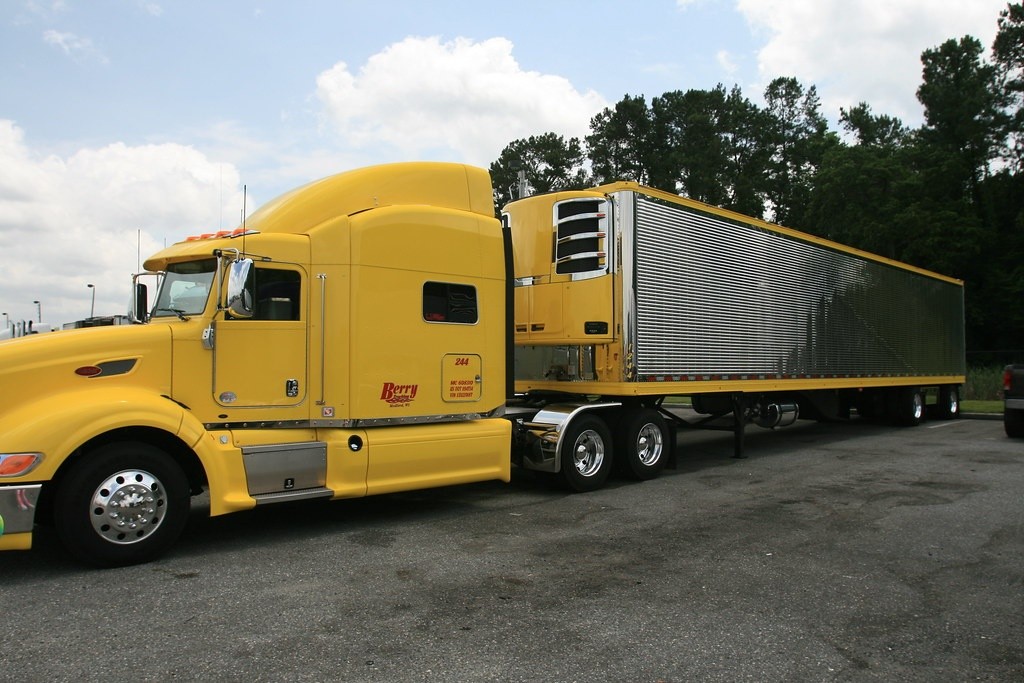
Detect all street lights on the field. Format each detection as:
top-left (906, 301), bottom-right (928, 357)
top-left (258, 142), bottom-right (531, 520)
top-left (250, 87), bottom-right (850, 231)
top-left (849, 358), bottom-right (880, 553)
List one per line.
top-left (2, 312), bottom-right (8, 328)
top-left (87, 284), bottom-right (95, 318)
top-left (34, 301), bottom-right (41, 323)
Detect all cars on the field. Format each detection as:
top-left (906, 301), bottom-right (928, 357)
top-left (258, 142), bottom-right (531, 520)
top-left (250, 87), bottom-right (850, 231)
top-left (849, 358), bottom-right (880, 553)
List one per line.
top-left (1002, 365), bottom-right (1024, 438)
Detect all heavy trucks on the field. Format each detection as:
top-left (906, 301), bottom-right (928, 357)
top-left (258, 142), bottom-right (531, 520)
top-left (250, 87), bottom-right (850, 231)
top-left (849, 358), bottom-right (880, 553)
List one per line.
top-left (0, 164), bottom-right (967, 566)
